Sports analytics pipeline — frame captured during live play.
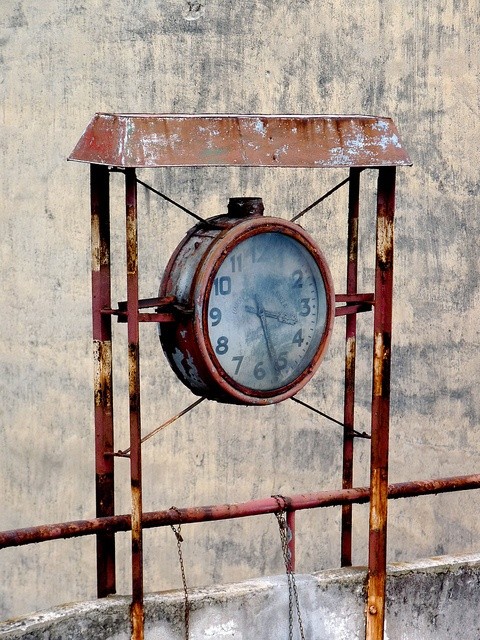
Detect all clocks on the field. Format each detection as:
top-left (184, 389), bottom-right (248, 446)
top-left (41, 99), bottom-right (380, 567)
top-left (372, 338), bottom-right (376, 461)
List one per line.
top-left (157, 197), bottom-right (336, 405)
